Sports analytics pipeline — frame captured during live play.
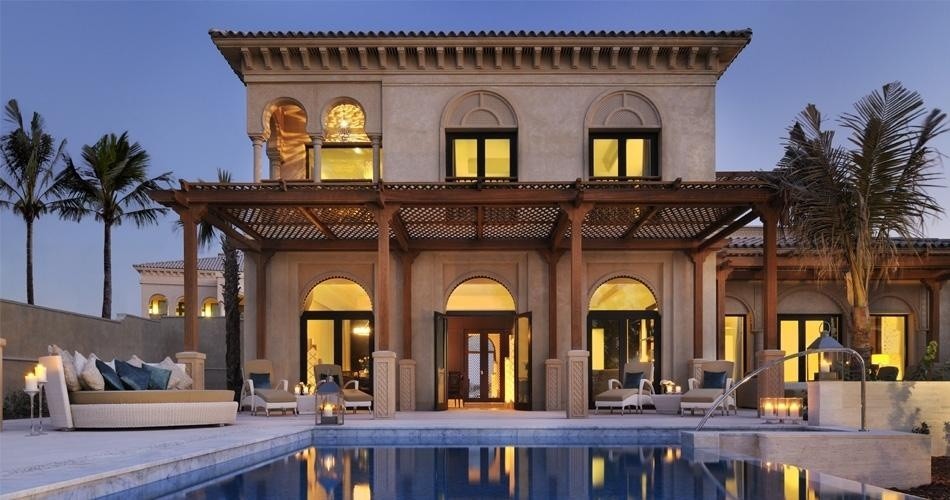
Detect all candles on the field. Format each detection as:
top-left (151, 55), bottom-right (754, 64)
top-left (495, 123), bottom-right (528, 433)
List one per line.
top-left (25, 365), bottom-right (48, 393)
top-left (764, 405), bottom-right (799, 418)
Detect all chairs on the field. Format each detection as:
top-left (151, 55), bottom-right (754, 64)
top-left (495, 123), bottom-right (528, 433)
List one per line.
top-left (812, 362), bottom-right (900, 382)
top-left (595, 358), bottom-right (735, 417)
top-left (240, 358), bottom-right (373, 419)
top-left (446, 369), bottom-right (466, 411)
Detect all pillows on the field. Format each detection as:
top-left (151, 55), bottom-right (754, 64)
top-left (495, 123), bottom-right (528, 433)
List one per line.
top-left (50, 341), bottom-right (196, 392)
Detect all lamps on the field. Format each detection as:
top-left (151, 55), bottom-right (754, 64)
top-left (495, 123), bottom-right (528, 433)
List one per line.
top-left (807, 320), bottom-right (843, 353)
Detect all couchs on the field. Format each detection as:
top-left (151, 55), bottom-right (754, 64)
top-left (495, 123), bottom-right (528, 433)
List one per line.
top-left (36, 352), bottom-right (237, 427)
